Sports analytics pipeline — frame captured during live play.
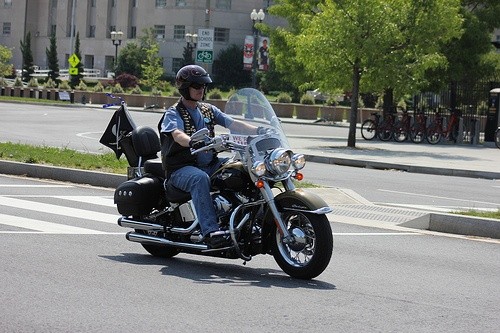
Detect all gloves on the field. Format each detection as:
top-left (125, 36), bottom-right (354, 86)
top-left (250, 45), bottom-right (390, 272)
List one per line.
top-left (257, 127), bottom-right (274, 135)
top-left (189, 140), bottom-right (205, 150)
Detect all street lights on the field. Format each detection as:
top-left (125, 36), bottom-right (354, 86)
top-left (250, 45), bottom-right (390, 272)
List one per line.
top-left (110, 28), bottom-right (124, 87)
top-left (249, 8), bottom-right (264, 94)
top-left (186, 31), bottom-right (199, 65)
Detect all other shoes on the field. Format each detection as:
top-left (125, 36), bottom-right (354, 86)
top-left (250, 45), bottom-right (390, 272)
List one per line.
top-left (206, 235), bottom-right (230, 250)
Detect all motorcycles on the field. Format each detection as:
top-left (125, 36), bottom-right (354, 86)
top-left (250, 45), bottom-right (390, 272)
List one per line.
top-left (114, 88), bottom-right (333, 280)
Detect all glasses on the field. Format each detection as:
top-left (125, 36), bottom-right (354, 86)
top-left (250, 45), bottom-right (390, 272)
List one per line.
top-left (191, 82), bottom-right (206, 90)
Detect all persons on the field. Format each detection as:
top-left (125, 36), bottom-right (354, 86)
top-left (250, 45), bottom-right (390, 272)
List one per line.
top-left (259, 39), bottom-right (270, 64)
top-left (157, 65), bottom-right (277, 248)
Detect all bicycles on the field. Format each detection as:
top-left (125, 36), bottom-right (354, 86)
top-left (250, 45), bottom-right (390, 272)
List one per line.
top-left (361, 104), bottom-right (467, 146)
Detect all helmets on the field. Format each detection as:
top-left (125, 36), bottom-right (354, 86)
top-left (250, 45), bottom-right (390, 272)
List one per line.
top-left (175, 65), bottom-right (212, 90)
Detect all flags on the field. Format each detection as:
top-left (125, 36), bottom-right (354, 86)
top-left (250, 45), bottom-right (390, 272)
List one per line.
top-left (98, 104), bottom-right (137, 160)
